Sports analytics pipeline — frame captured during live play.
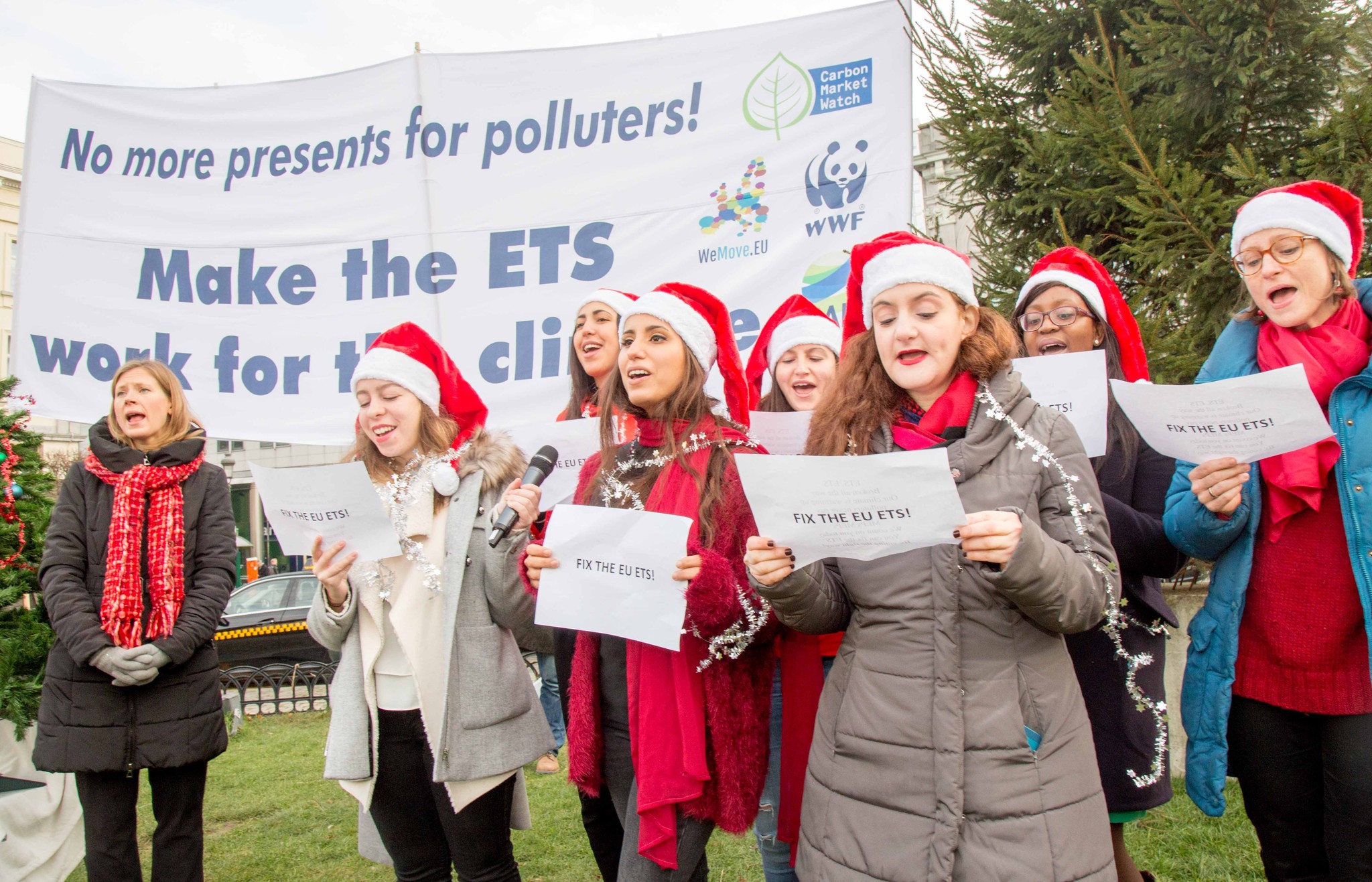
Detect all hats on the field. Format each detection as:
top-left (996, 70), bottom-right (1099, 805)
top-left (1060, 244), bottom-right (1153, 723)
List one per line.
top-left (840, 231), bottom-right (980, 365)
top-left (746, 294), bottom-right (843, 412)
top-left (1015, 246), bottom-right (1152, 384)
top-left (573, 288), bottom-right (641, 316)
top-left (350, 322), bottom-right (488, 496)
top-left (619, 282), bottom-right (749, 436)
top-left (1230, 180), bottom-right (1364, 280)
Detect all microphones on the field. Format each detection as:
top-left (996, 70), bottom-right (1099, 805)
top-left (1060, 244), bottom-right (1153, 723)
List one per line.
top-left (486, 444), bottom-right (559, 549)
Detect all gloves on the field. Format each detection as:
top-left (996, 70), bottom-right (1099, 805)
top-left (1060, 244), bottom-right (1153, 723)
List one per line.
top-left (89, 643), bottom-right (172, 686)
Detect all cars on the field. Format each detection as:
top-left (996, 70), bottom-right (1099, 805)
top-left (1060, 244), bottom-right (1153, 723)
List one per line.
top-left (213, 569), bottom-right (341, 681)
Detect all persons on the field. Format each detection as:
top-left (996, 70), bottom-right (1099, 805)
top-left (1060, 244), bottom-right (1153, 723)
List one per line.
top-left (1162, 177), bottom-right (1372, 882)
top-left (516, 283), bottom-right (782, 880)
top-left (745, 230), bottom-right (1121, 881)
top-left (525, 288), bottom-right (711, 880)
top-left (269, 558), bottom-right (279, 575)
top-left (305, 320), bottom-right (557, 882)
top-left (257, 560), bottom-right (270, 579)
top-left (992, 245), bottom-right (1194, 882)
top-left (31, 357), bottom-right (242, 882)
top-left (734, 293), bottom-right (847, 882)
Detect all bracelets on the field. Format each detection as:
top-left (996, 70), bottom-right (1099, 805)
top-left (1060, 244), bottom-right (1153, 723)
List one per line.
top-left (327, 599), bottom-right (345, 608)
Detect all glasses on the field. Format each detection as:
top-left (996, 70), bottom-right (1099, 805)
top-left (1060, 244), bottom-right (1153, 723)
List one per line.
top-left (1017, 306), bottom-right (1097, 332)
top-left (1229, 236), bottom-right (1319, 276)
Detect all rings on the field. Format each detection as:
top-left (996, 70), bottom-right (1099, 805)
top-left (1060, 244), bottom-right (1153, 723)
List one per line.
top-left (1207, 487), bottom-right (1219, 500)
